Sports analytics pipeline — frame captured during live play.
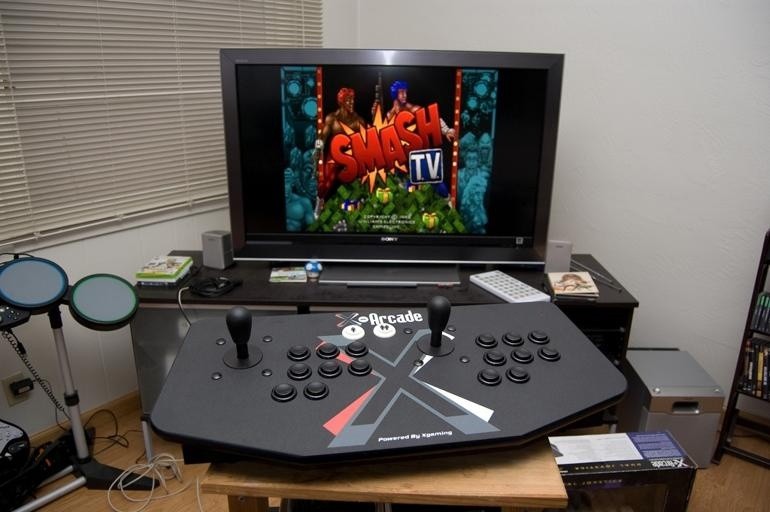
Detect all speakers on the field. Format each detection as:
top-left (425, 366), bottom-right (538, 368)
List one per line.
top-left (616, 350), bottom-right (726, 470)
top-left (202, 231), bottom-right (232, 271)
top-left (544, 239), bottom-right (573, 274)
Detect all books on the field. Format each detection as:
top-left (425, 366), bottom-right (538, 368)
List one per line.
top-left (546, 270), bottom-right (599, 302)
top-left (135, 252), bottom-right (194, 289)
top-left (269, 265), bottom-right (308, 284)
top-left (735, 292), bottom-right (769, 402)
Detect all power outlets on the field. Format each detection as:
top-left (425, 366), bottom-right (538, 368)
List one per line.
top-left (0, 369), bottom-right (30, 407)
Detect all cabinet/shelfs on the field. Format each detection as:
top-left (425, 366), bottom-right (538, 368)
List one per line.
top-left (711, 231), bottom-right (770, 469)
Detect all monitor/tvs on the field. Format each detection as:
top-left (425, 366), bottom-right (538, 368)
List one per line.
top-left (220, 47), bottom-right (565, 288)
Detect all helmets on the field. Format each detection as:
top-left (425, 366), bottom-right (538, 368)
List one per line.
top-left (390, 81), bottom-right (408, 99)
top-left (337, 88), bottom-right (355, 105)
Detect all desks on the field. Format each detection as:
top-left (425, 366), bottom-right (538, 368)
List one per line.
top-left (199, 435), bottom-right (569, 511)
top-left (129, 250), bottom-right (640, 465)
